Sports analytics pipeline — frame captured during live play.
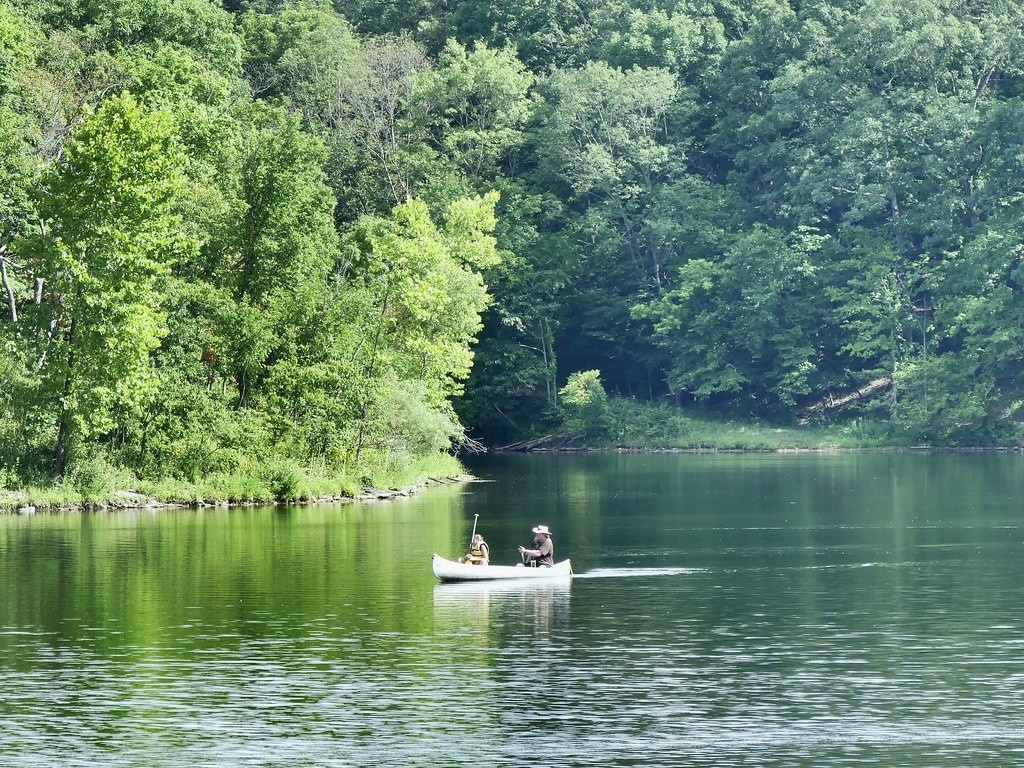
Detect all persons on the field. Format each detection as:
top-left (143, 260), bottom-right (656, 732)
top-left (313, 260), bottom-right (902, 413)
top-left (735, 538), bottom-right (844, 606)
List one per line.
top-left (459, 534), bottom-right (489, 565)
top-left (515, 525), bottom-right (554, 568)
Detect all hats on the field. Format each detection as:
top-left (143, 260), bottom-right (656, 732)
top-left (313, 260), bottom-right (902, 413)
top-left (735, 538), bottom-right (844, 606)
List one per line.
top-left (532, 525), bottom-right (553, 535)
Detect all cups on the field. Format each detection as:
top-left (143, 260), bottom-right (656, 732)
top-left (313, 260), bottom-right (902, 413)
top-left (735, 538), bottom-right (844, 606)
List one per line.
top-left (531, 559), bottom-right (536, 567)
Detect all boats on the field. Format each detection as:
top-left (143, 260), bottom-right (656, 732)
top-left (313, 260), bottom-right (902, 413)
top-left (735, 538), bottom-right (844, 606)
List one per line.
top-left (433, 576), bottom-right (572, 596)
top-left (433, 554), bottom-right (572, 581)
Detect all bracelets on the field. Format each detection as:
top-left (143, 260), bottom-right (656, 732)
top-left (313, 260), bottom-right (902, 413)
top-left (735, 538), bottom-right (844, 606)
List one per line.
top-left (524, 551), bottom-right (526, 554)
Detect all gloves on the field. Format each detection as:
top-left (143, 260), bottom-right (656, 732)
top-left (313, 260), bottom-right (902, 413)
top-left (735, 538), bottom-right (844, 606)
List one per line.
top-left (466, 554), bottom-right (472, 559)
top-left (518, 546), bottom-right (526, 553)
